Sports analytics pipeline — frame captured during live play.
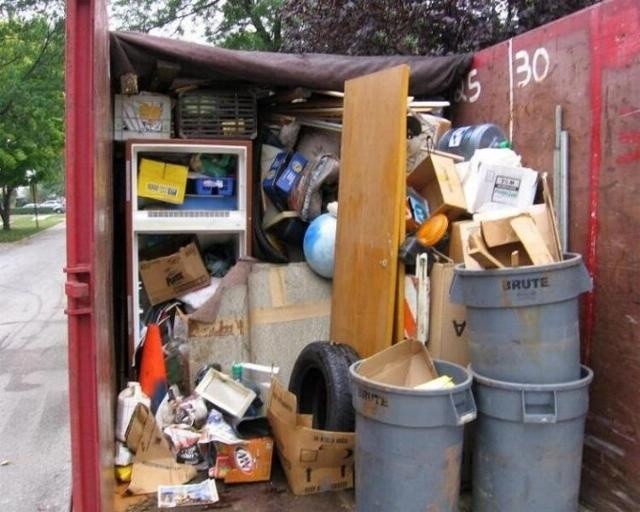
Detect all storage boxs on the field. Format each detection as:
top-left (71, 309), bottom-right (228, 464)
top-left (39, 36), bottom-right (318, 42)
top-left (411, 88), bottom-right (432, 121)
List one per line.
top-left (406, 153), bottom-right (467, 222)
top-left (137, 158), bottom-right (189, 205)
top-left (480, 212), bottom-right (556, 271)
top-left (356, 338), bottom-right (439, 388)
top-left (428, 264), bottom-right (470, 367)
top-left (209, 437), bottom-right (273, 484)
top-left (138, 234), bottom-right (210, 306)
top-left (265, 379), bottom-right (355, 496)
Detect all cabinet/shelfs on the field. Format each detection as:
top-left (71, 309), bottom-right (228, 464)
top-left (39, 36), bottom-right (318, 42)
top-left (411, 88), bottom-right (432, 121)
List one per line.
top-left (125, 139), bottom-right (253, 382)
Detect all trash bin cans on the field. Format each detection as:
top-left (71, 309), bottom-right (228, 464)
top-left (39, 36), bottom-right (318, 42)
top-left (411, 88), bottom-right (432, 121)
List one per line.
top-left (467, 362), bottom-right (595, 512)
top-left (348, 356), bottom-right (477, 511)
top-left (448, 252), bottom-right (591, 384)
